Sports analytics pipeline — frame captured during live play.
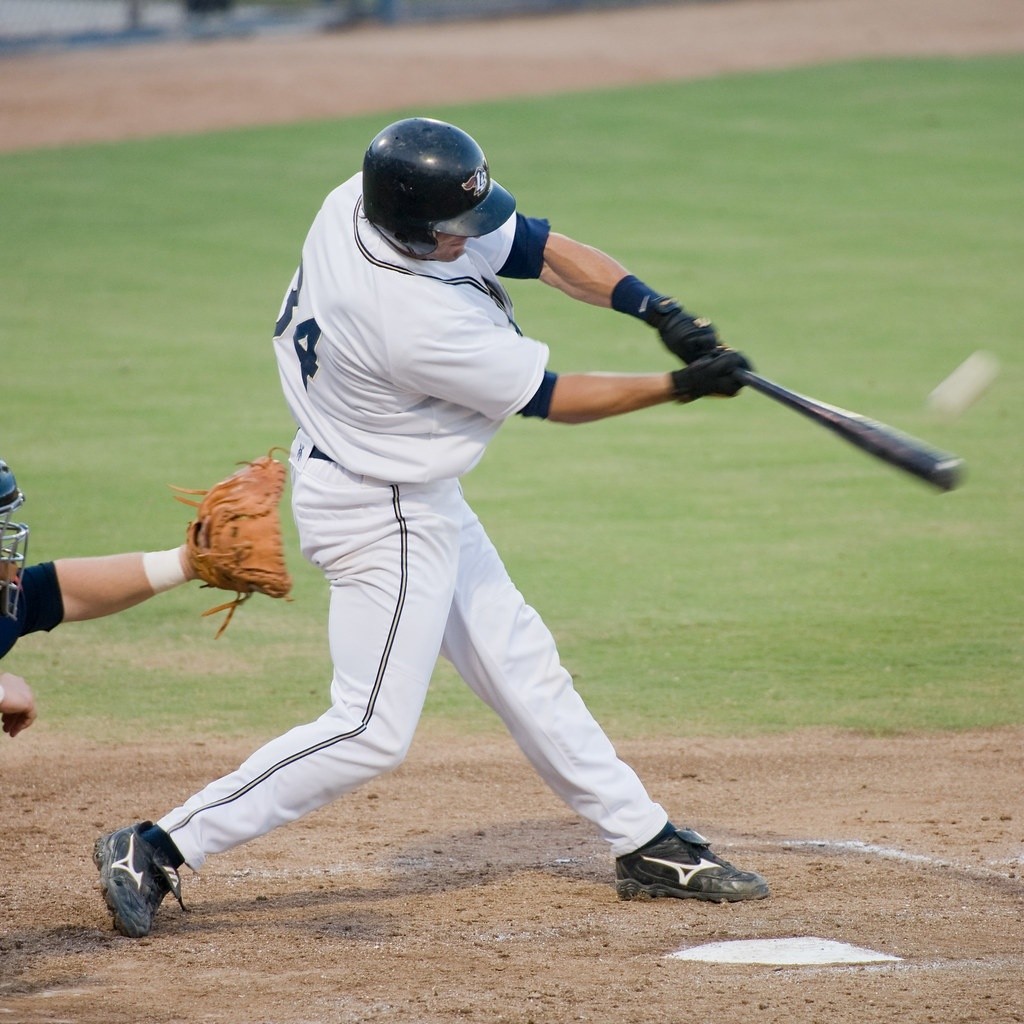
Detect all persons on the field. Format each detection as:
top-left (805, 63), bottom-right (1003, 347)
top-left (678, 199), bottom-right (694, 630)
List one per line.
top-left (92, 116), bottom-right (769, 940)
top-left (0, 457), bottom-right (294, 739)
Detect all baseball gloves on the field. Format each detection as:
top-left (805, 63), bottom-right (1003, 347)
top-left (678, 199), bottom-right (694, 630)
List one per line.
top-left (167, 442), bottom-right (296, 642)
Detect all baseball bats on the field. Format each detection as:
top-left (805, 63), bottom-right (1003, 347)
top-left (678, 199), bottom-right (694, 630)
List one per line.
top-left (735, 366), bottom-right (967, 492)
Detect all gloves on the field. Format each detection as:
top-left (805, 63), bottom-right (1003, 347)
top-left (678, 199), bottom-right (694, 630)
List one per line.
top-left (647, 296), bottom-right (719, 365)
top-left (672, 344), bottom-right (752, 406)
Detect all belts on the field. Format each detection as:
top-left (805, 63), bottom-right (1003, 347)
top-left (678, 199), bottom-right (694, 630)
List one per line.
top-left (309, 446), bottom-right (335, 463)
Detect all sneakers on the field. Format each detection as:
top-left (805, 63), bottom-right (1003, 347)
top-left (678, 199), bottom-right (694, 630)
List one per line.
top-left (615, 827), bottom-right (771, 902)
top-left (93, 821), bottom-right (187, 937)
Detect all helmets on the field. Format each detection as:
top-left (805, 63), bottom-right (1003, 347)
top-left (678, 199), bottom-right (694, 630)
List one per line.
top-left (362, 117), bottom-right (516, 256)
top-left (0, 461), bottom-right (29, 624)
